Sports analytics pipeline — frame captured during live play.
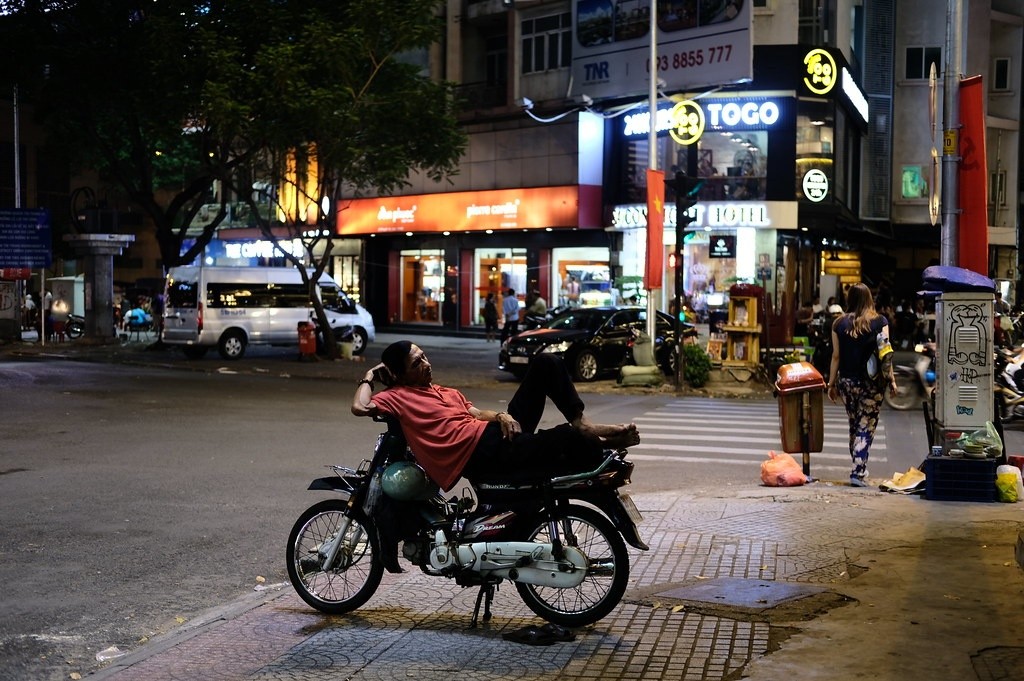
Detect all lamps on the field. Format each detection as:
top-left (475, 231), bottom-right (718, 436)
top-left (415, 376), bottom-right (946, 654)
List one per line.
top-left (574, 94), bottom-right (650, 120)
top-left (513, 96), bottom-right (579, 123)
top-left (656, 78), bottom-right (736, 104)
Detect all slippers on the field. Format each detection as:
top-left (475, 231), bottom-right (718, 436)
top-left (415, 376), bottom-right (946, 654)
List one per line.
top-left (502, 624), bottom-right (553, 646)
top-left (540, 623), bottom-right (577, 642)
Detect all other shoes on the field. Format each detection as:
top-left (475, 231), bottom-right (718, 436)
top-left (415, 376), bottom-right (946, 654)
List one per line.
top-left (850, 478), bottom-right (867, 487)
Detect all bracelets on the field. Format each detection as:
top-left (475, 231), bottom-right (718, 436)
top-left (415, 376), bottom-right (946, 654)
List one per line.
top-left (828, 382), bottom-right (835, 388)
top-left (358, 379), bottom-right (374, 391)
top-left (495, 413), bottom-right (505, 418)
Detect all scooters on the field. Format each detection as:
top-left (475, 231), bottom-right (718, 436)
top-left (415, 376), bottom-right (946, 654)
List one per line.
top-left (65, 313), bottom-right (85, 340)
top-left (882, 310), bottom-right (1024, 425)
top-left (523, 304), bottom-right (572, 331)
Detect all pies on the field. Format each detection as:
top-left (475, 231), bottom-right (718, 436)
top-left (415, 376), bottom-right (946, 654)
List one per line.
top-left (964, 438), bottom-right (1000, 456)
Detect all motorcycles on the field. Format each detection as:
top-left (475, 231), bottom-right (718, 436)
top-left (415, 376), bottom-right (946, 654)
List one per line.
top-left (286, 414), bottom-right (651, 631)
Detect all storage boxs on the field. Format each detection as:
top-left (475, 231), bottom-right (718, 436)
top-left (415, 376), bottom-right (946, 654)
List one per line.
top-left (924, 455), bottom-right (999, 502)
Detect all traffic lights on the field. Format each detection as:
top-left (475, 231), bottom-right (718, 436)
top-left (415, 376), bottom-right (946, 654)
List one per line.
top-left (668, 252), bottom-right (678, 269)
top-left (674, 172), bottom-right (704, 245)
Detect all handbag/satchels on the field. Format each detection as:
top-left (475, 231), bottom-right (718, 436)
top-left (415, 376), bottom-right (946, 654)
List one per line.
top-left (867, 315), bottom-right (893, 386)
top-left (995, 465), bottom-right (1023, 503)
top-left (957, 421), bottom-right (1003, 457)
top-left (1000, 313), bottom-right (1014, 331)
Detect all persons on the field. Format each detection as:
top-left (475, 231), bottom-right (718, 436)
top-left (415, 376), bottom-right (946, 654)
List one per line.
top-left (994, 293), bottom-right (1012, 345)
top-left (501, 289), bottom-right (519, 347)
top-left (45, 288), bottom-right (53, 340)
top-left (131, 304), bottom-right (149, 332)
top-left (524, 290), bottom-right (546, 314)
top-left (701, 160), bottom-right (713, 176)
top-left (810, 286), bottom-right (845, 393)
top-left (484, 293), bottom-right (497, 342)
top-left (827, 282), bottom-right (898, 487)
top-left (671, 165), bottom-right (687, 179)
top-left (567, 277), bottom-right (580, 304)
top-left (352, 340), bottom-right (640, 492)
top-left (885, 298), bottom-right (934, 351)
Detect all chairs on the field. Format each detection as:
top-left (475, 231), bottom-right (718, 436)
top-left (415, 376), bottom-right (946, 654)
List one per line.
top-left (128, 317), bottom-right (150, 344)
top-left (34, 320), bottom-right (71, 344)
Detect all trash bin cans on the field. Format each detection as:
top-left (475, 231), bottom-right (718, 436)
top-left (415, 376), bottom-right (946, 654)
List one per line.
top-left (297, 325), bottom-right (317, 354)
top-left (776, 362), bottom-right (827, 453)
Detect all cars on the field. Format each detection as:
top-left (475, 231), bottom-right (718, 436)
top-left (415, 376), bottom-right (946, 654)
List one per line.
top-left (497, 304), bottom-right (700, 383)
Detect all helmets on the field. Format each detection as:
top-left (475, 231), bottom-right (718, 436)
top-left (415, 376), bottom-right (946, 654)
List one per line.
top-left (382, 463), bottom-right (428, 501)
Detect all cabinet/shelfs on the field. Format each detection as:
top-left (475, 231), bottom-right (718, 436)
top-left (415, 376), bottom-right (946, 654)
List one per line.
top-left (721, 296), bottom-right (762, 367)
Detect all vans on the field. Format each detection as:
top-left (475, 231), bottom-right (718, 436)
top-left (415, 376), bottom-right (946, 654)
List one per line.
top-left (161, 264), bottom-right (376, 362)
